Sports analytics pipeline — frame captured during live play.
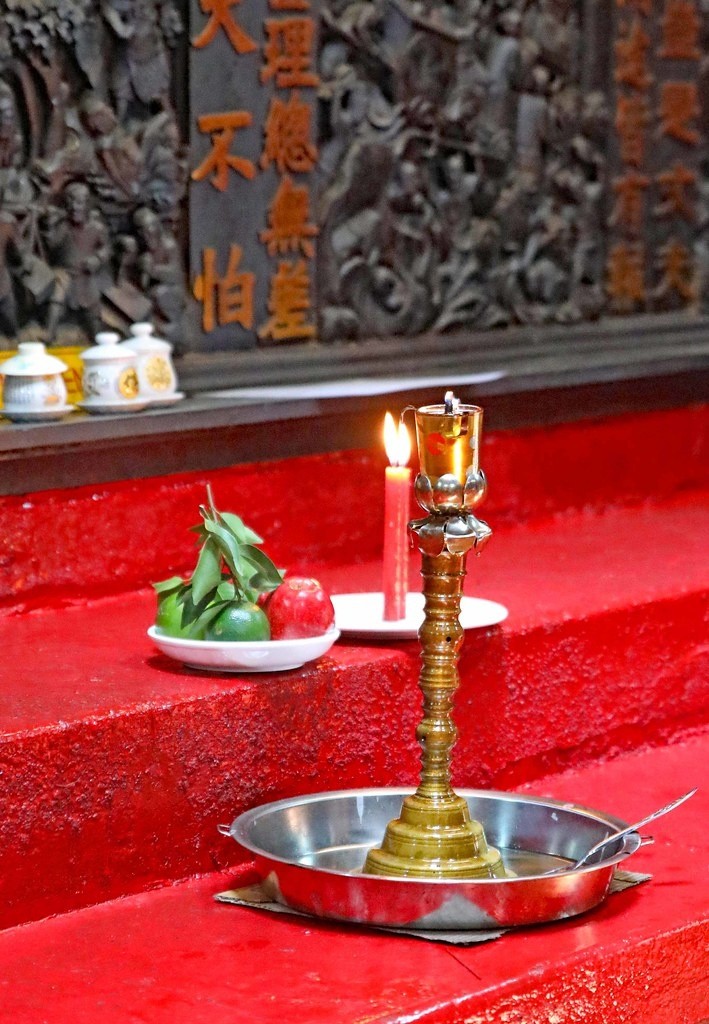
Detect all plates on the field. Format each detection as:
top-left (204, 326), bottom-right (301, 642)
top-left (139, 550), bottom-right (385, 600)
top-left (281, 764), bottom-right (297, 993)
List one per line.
top-left (0, 392), bottom-right (185, 421)
top-left (328, 592), bottom-right (509, 641)
top-left (146, 625), bottom-right (340, 673)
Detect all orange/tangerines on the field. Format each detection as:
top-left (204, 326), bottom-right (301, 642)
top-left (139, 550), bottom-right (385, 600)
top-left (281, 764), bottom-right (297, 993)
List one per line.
top-left (204, 600), bottom-right (270, 641)
top-left (155, 593), bottom-right (215, 640)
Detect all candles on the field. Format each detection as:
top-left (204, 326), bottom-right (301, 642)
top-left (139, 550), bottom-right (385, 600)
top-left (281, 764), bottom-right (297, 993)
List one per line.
top-left (415, 390), bottom-right (485, 476)
top-left (383, 407), bottom-right (411, 621)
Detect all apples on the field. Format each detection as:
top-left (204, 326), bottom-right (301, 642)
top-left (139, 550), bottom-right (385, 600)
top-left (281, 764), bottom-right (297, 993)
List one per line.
top-left (258, 577), bottom-right (334, 640)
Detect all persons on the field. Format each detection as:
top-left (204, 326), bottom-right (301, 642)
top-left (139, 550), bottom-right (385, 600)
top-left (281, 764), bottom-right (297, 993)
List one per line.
top-left (118, 207), bottom-right (186, 320)
top-left (37, 182), bottom-right (112, 348)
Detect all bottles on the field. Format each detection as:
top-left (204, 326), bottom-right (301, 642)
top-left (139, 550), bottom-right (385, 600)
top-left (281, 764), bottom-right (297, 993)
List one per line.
top-left (0, 321), bottom-right (177, 410)
